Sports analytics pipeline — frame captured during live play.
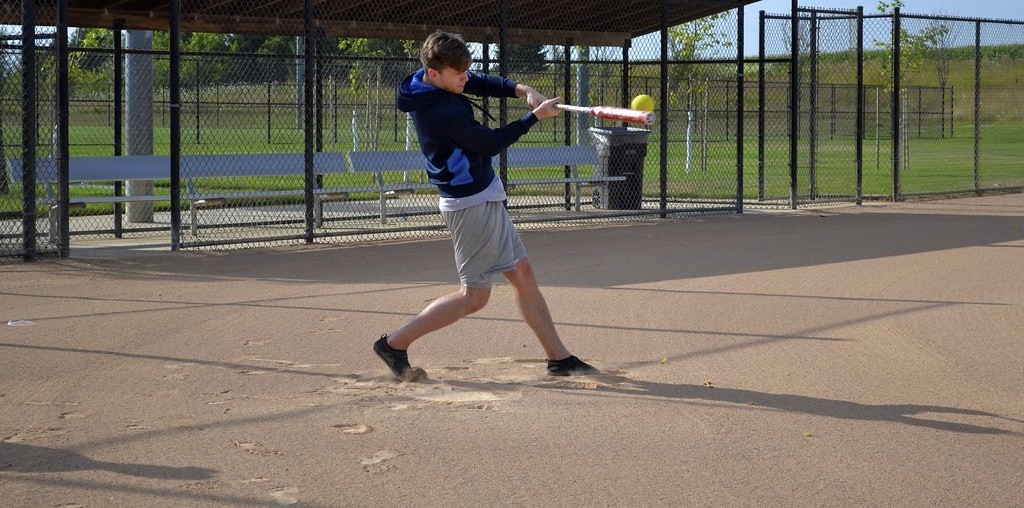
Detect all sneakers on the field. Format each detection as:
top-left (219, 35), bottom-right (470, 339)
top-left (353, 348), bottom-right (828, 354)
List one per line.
top-left (547, 356), bottom-right (599, 377)
top-left (373, 334), bottom-right (427, 381)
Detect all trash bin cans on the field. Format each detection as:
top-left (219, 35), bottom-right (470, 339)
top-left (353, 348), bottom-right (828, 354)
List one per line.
top-left (588, 126), bottom-right (653, 210)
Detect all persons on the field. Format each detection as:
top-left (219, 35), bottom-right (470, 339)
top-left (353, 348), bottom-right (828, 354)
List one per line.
top-left (373, 31), bottom-right (598, 382)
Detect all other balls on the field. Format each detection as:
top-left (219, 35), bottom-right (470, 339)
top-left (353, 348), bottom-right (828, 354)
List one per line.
top-left (632, 93), bottom-right (656, 110)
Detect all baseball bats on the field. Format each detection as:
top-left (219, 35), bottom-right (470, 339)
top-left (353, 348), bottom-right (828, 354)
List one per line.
top-left (549, 103), bottom-right (657, 125)
